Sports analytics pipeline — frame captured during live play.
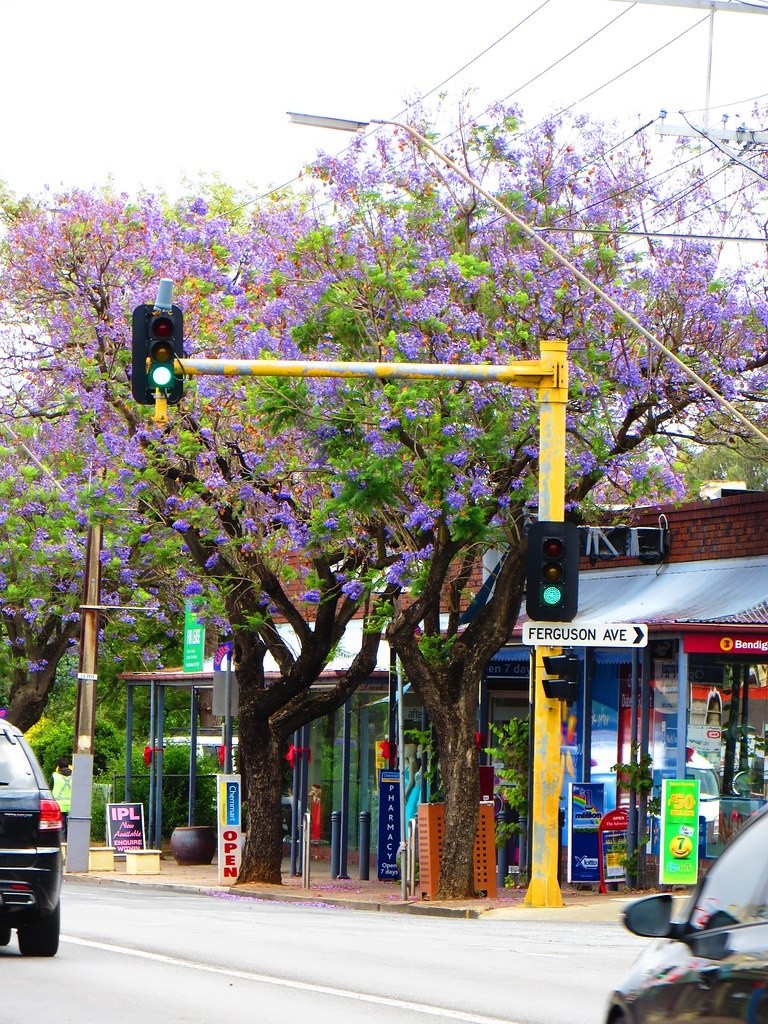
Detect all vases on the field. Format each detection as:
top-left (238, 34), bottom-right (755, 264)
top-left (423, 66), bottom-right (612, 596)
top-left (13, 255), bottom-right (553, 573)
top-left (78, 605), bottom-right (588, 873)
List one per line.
top-left (170, 826), bottom-right (216, 866)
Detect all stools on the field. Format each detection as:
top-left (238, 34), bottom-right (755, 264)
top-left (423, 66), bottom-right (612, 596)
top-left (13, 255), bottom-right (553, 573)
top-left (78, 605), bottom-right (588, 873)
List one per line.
top-left (124, 849), bottom-right (162, 874)
top-left (88, 847), bottom-right (116, 871)
top-left (61, 842), bottom-right (67, 860)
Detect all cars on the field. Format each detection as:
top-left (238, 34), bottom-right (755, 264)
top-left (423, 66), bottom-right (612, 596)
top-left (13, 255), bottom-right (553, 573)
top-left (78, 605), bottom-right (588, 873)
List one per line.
top-left (0, 718), bottom-right (65, 958)
top-left (605, 802), bottom-right (768, 1024)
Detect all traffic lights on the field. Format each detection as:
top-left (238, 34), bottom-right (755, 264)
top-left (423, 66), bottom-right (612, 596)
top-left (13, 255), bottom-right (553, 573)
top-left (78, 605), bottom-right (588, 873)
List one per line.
top-left (132, 302), bottom-right (186, 408)
top-left (525, 521), bottom-right (580, 622)
top-left (539, 650), bottom-right (580, 701)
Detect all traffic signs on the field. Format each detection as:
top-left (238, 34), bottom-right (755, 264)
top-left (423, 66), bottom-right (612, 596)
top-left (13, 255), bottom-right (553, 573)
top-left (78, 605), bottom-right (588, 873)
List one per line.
top-left (521, 622), bottom-right (650, 648)
